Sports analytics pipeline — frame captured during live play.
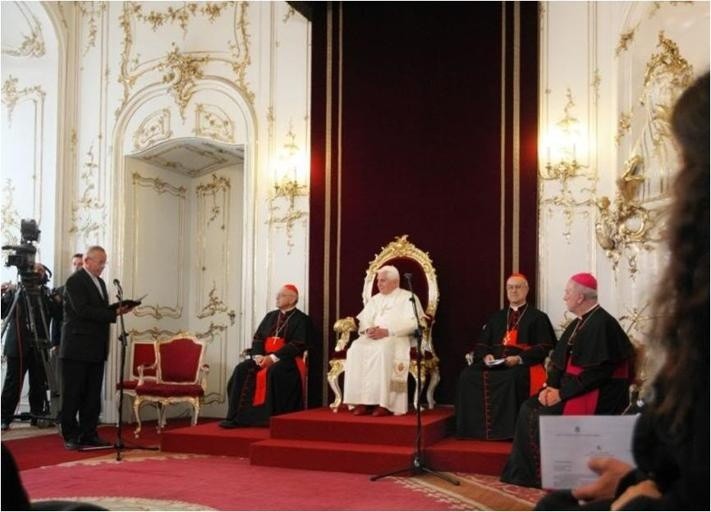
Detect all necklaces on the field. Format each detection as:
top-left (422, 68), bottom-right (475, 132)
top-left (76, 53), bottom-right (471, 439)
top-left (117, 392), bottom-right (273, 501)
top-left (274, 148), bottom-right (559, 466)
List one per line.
top-left (501, 302), bottom-right (530, 348)
top-left (567, 306), bottom-right (601, 355)
top-left (272, 310), bottom-right (298, 345)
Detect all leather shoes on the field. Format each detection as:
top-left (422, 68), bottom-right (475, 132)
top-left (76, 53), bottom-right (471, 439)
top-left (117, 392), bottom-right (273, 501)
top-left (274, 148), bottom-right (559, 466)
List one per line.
top-left (65, 438), bottom-right (78, 449)
top-left (372, 406), bottom-right (387, 416)
top-left (220, 420), bottom-right (237, 427)
top-left (1, 422), bottom-right (9, 430)
top-left (354, 406), bottom-right (368, 416)
top-left (80, 437), bottom-right (110, 446)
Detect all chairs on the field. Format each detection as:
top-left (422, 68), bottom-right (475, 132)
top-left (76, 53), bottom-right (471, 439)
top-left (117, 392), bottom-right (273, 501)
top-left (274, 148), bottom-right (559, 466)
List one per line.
top-left (114, 327), bottom-right (163, 429)
top-left (132, 328), bottom-right (206, 440)
top-left (325, 234), bottom-right (442, 416)
top-left (541, 333), bottom-right (640, 421)
top-left (239, 346), bottom-right (308, 410)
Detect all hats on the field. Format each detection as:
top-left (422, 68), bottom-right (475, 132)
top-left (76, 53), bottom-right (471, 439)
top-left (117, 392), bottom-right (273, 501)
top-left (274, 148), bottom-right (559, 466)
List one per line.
top-left (571, 273), bottom-right (597, 289)
top-left (285, 285), bottom-right (298, 295)
top-left (509, 273), bottom-right (528, 285)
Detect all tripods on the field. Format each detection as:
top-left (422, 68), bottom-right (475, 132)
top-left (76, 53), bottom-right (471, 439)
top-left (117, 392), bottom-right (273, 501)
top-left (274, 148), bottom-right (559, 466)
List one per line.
top-left (79, 335), bottom-right (160, 461)
top-left (369, 329), bottom-right (460, 486)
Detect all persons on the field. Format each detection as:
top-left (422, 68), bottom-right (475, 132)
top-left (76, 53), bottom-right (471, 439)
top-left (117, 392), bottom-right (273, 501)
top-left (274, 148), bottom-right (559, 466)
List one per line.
top-left (455, 271), bottom-right (555, 440)
top-left (498, 273), bottom-right (636, 489)
top-left (50, 254), bottom-right (84, 425)
top-left (58, 245), bottom-right (133, 450)
top-left (535, 72), bottom-right (710, 510)
top-left (218, 285), bottom-right (323, 428)
top-left (341, 265), bottom-right (424, 416)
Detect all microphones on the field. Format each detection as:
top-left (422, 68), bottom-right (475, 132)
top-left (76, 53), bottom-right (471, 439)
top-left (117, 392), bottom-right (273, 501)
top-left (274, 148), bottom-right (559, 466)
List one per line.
top-left (114, 278), bottom-right (123, 295)
